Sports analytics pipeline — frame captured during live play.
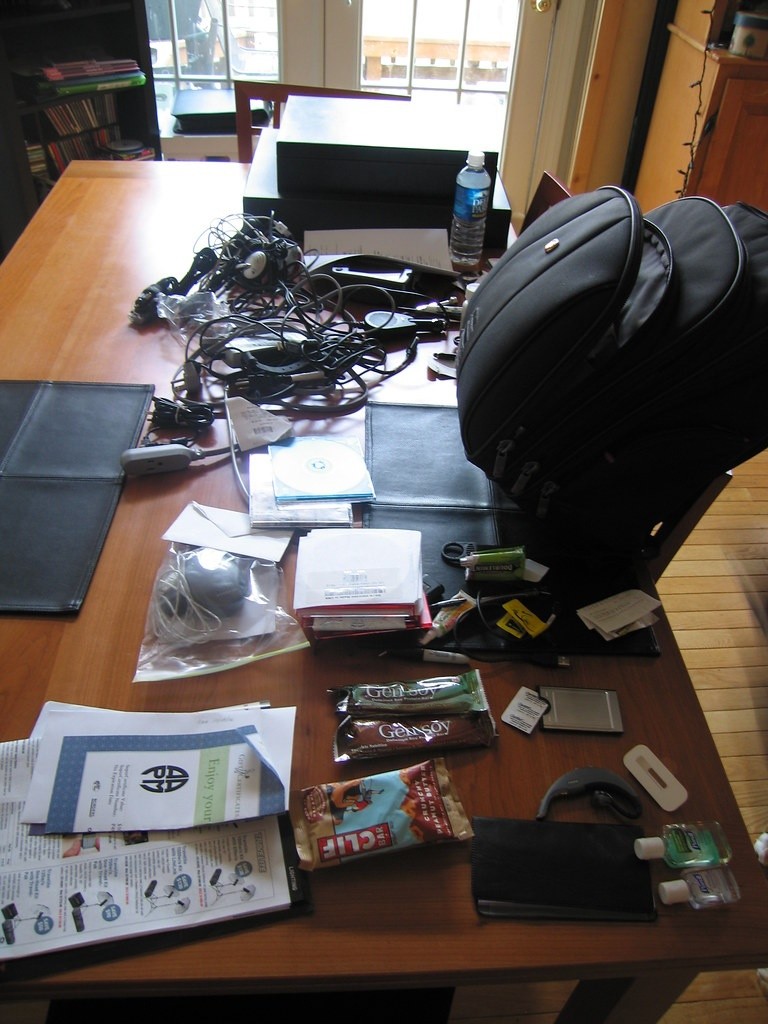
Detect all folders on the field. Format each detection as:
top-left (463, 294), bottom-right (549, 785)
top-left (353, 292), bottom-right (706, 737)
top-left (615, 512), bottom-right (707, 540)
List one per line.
top-left (54, 75), bottom-right (146, 97)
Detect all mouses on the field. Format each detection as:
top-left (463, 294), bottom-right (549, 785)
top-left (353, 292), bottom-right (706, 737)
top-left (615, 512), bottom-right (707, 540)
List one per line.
top-left (185, 555), bottom-right (244, 618)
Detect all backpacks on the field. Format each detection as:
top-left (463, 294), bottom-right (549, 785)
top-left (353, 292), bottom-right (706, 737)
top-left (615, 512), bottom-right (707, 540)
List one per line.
top-left (453, 185), bottom-right (768, 538)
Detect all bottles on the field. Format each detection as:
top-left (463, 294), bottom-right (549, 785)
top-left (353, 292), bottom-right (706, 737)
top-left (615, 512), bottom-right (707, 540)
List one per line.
top-left (658, 864), bottom-right (741, 910)
top-left (634, 822), bottom-right (731, 870)
top-left (450, 151), bottom-right (491, 265)
top-left (460, 283), bottom-right (480, 329)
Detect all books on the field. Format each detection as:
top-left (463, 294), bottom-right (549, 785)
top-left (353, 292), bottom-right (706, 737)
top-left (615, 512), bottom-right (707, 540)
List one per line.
top-left (471, 814), bottom-right (658, 921)
top-left (14, 51), bottom-right (158, 184)
top-left (248, 453), bottom-right (432, 639)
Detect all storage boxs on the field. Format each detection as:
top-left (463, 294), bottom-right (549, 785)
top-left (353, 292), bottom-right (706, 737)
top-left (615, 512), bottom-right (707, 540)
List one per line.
top-left (242, 128), bottom-right (511, 257)
top-left (276, 94), bottom-right (500, 206)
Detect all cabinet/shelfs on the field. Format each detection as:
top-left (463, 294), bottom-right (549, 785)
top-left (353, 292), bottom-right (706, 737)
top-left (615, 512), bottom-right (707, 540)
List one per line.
top-left (0, 0), bottom-right (163, 265)
top-left (632, 20), bottom-right (768, 215)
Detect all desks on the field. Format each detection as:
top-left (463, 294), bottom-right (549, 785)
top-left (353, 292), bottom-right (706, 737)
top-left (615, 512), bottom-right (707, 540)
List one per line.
top-left (0, 159), bottom-right (768, 1024)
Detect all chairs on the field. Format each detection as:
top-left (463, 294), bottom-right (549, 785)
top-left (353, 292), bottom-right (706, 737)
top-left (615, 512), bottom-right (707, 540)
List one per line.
top-left (231, 78), bottom-right (412, 163)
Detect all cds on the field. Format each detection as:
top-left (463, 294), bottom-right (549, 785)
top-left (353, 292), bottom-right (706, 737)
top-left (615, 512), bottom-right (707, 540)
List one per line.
top-left (273, 439), bottom-right (367, 494)
